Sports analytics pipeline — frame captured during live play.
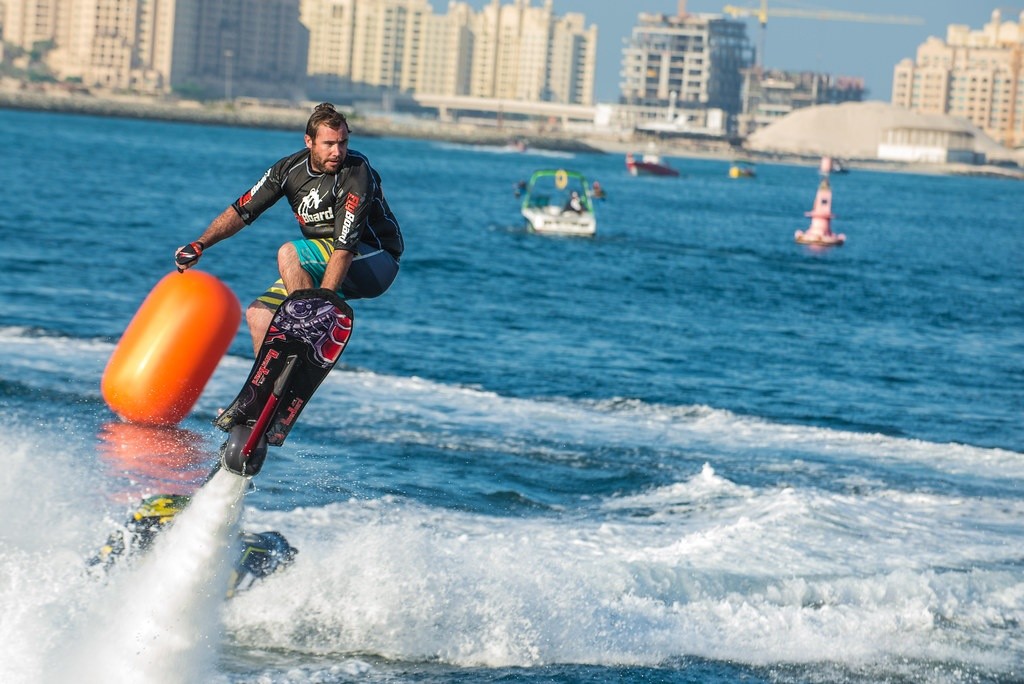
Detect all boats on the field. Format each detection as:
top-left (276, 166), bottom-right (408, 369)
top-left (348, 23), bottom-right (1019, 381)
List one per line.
top-left (728, 160), bottom-right (757, 179)
top-left (514, 168), bottom-right (607, 236)
top-left (625, 142), bottom-right (679, 177)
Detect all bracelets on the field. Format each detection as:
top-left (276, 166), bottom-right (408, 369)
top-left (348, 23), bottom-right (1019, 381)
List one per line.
top-left (195, 241), bottom-right (204, 252)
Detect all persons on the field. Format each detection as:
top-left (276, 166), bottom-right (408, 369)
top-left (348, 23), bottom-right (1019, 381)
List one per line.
top-left (565, 190), bottom-right (589, 215)
top-left (176, 103), bottom-right (404, 416)
